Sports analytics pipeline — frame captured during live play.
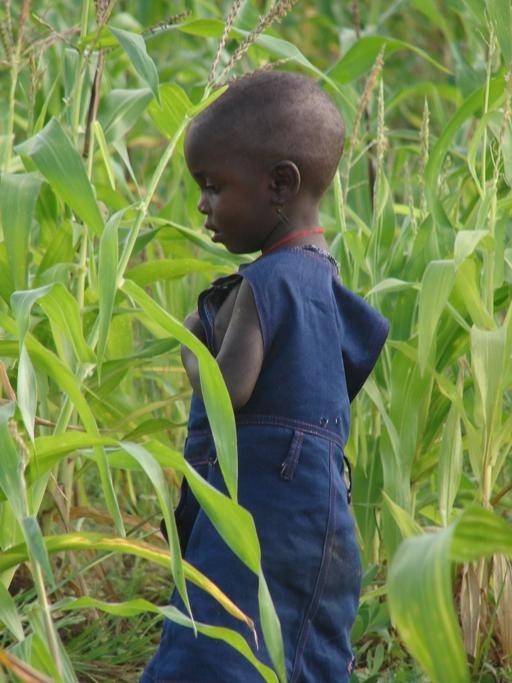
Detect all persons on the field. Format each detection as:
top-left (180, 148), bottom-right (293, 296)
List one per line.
top-left (137, 67), bottom-right (390, 682)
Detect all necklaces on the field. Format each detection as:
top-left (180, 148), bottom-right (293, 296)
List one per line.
top-left (260, 226), bottom-right (325, 254)
